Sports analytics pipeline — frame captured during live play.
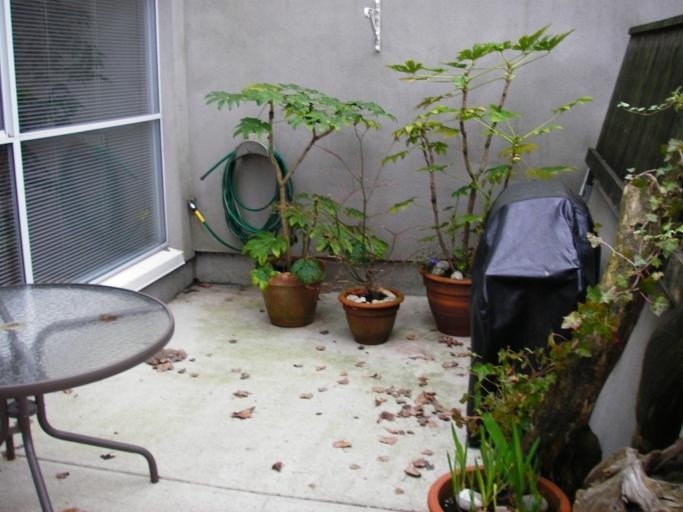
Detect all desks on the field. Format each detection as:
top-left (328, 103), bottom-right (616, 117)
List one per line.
top-left (0, 283), bottom-right (175, 512)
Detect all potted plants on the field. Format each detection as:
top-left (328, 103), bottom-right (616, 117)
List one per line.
top-left (427, 411), bottom-right (574, 510)
top-left (308, 119), bottom-right (421, 345)
top-left (206, 80), bottom-right (391, 331)
top-left (396, 30), bottom-right (587, 338)
